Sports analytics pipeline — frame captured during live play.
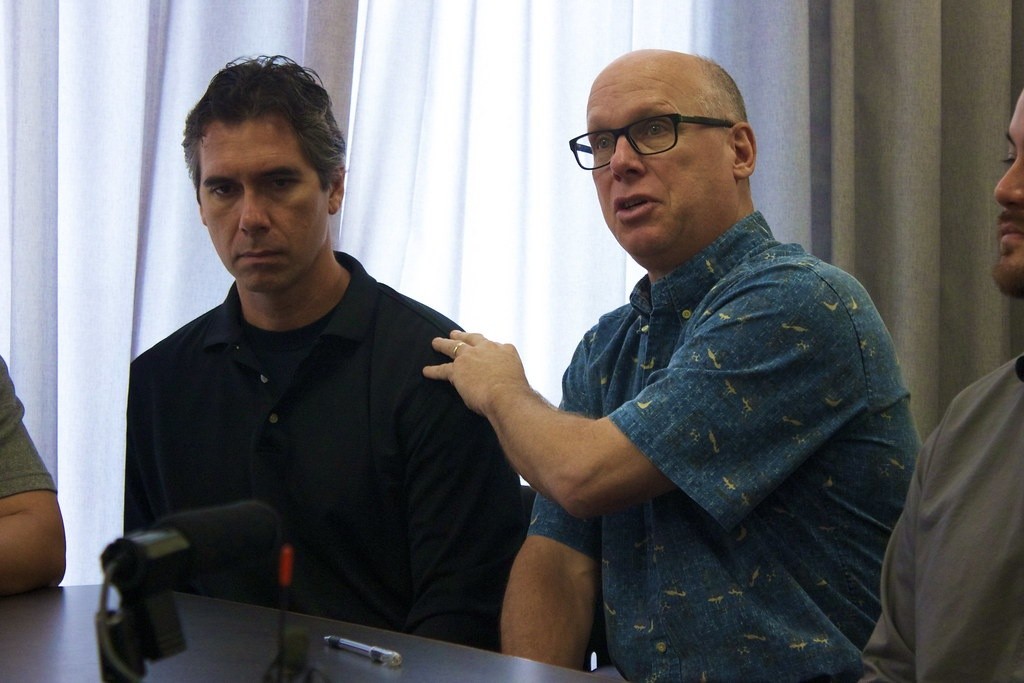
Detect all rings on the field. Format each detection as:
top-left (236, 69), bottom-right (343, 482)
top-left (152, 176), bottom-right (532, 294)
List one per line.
top-left (453, 342), bottom-right (465, 358)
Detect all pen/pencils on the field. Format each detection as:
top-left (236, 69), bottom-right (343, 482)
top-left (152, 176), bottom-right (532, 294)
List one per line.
top-left (323, 635), bottom-right (403, 666)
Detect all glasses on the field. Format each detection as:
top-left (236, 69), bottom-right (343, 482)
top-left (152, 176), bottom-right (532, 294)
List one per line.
top-left (569, 112), bottom-right (735, 170)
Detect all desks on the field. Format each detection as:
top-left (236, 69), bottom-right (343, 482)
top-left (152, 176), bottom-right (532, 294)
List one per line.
top-left (0, 582), bottom-right (637, 683)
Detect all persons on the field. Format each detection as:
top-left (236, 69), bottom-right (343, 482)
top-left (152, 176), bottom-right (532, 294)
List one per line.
top-left (0, 351), bottom-right (68, 600)
top-left (123, 53), bottom-right (527, 654)
top-left (422, 51), bottom-right (920, 682)
top-left (860, 88), bottom-right (1023, 683)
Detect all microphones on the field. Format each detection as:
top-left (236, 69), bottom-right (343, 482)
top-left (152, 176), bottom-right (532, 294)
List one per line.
top-left (101, 501), bottom-right (282, 593)
